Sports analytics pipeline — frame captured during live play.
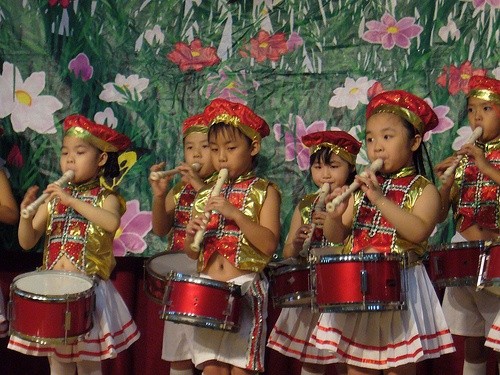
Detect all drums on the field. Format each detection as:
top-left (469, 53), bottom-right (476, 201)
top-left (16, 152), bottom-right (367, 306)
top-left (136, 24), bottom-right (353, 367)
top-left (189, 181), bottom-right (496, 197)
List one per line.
top-left (429, 240), bottom-right (500, 293)
top-left (143, 248), bottom-right (200, 304)
top-left (315, 252), bottom-right (408, 314)
top-left (7, 268), bottom-right (97, 346)
top-left (162, 270), bottom-right (242, 333)
top-left (270, 264), bottom-right (316, 307)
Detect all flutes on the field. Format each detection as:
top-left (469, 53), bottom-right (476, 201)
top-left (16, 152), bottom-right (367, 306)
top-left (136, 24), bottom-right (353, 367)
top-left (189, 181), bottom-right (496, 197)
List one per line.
top-left (326, 158), bottom-right (383, 213)
top-left (439, 125), bottom-right (483, 182)
top-left (20, 168), bottom-right (76, 219)
top-left (189, 168), bottom-right (229, 252)
top-left (299, 182), bottom-right (330, 258)
top-left (150, 162), bottom-right (201, 181)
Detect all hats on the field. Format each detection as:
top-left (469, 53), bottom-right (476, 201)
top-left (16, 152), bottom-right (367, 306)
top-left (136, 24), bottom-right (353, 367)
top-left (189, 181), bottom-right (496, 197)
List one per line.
top-left (464, 76), bottom-right (500, 104)
top-left (63, 114), bottom-right (131, 153)
top-left (301, 130), bottom-right (363, 166)
top-left (182, 114), bottom-right (210, 137)
top-left (367, 90), bottom-right (440, 139)
top-left (203, 98), bottom-right (270, 147)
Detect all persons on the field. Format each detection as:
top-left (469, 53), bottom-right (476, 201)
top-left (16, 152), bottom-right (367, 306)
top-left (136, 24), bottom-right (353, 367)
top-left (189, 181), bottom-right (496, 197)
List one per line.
top-left (6, 114), bottom-right (141, 375)
top-left (267, 130), bottom-right (362, 375)
top-left (0, 158), bottom-right (19, 341)
top-left (434, 76), bottom-right (500, 375)
top-left (184, 99), bottom-right (281, 375)
top-left (150, 115), bottom-right (214, 375)
top-left (309, 91), bottom-right (457, 375)
